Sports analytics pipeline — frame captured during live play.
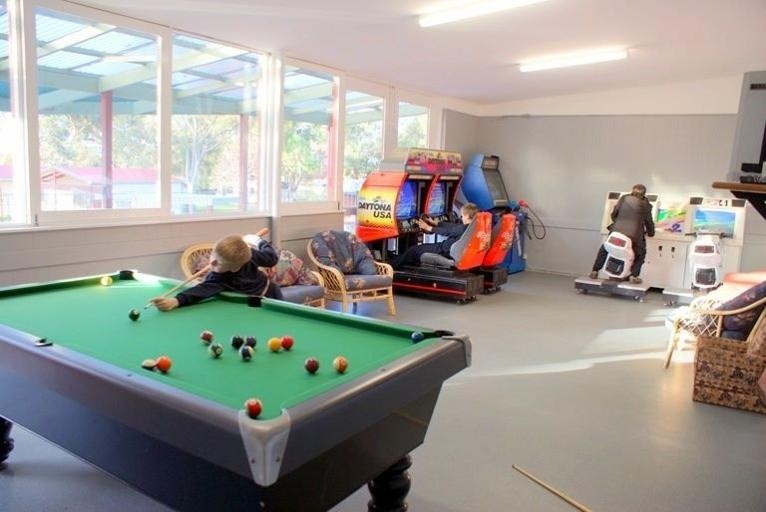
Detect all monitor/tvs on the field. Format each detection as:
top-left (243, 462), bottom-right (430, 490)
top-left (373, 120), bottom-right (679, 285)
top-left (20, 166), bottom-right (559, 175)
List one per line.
top-left (396, 181), bottom-right (418, 220)
top-left (428, 183), bottom-right (446, 215)
top-left (693, 208), bottom-right (738, 238)
top-left (484, 170), bottom-right (509, 202)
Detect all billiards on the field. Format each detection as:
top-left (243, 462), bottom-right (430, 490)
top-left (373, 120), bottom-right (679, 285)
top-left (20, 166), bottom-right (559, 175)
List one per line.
top-left (244, 399), bottom-right (263, 416)
top-left (200, 329), bottom-right (294, 360)
top-left (100, 276), bottom-right (113, 286)
top-left (130, 309), bottom-right (141, 320)
top-left (303, 358), bottom-right (319, 373)
top-left (334, 356), bottom-right (348, 373)
top-left (411, 331), bottom-right (424, 343)
top-left (156, 356), bottom-right (172, 372)
top-left (142, 359), bottom-right (155, 370)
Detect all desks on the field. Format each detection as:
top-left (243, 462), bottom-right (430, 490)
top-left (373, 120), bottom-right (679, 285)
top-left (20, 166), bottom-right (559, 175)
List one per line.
top-left (0, 267), bottom-right (474, 512)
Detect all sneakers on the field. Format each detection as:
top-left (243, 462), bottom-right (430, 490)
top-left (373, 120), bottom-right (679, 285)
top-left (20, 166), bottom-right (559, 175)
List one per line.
top-left (588, 271), bottom-right (598, 279)
top-left (629, 275), bottom-right (642, 283)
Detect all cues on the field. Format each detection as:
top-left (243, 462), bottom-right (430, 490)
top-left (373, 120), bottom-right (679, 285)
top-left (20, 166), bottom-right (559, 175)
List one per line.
top-left (510, 462), bottom-right (589, 511)
top-left (144, 228), bottom-right (269, 309)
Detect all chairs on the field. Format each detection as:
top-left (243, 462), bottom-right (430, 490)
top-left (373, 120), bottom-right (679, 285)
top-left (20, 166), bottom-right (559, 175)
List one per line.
top-left (420, 211), bottom-right (493, 277)
top-left (307, 225), bottom-right (398, 316)
top-left (690, 308), bottom-right (766, 414)
top-left (483, 212), bottom-right (518, 272)
top-left (179, 240), bottom-right (326, 311)
top-left (663, 279), bottom-right (766, 368)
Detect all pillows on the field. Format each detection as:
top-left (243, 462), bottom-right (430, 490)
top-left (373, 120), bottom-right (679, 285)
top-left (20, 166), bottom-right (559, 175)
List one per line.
top-left (262, 248), bottom-right (304, 287)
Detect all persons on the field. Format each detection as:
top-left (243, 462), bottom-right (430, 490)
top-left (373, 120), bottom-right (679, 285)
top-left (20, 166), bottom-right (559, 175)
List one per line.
top-left (389, 202), bottom-right (478, 271)
top-left (589, 184), bottom-right (654, 284)
top-left (149, 232), bottom-right (284, 311)
